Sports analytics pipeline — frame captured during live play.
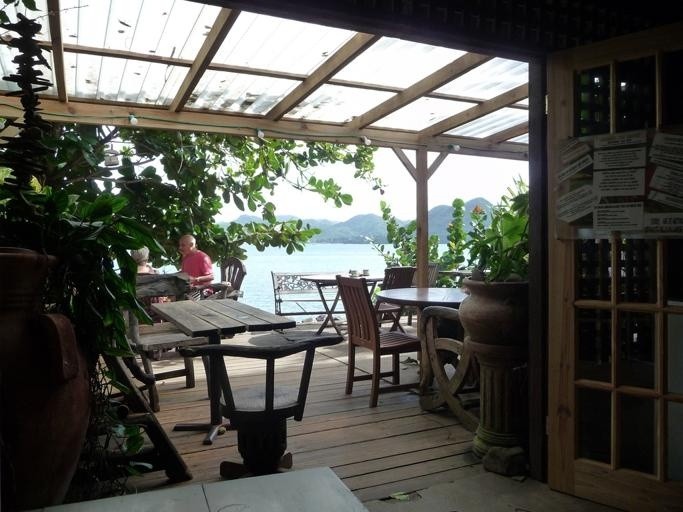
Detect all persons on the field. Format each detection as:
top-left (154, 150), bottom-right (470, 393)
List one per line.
top-left (130, 244), bottom-right (171, 323)
top-left (179, 234), bottom-right (214, 302)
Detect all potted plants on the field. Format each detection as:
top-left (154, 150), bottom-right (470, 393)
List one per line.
top-left (458, 192), bottom-right (530, 458)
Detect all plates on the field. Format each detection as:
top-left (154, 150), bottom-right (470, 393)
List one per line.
top-left (349, 276), bottom-right (359, 277)
top-left (360, 275), bottom-right (370, 277)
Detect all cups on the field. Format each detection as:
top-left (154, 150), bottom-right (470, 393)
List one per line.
top-left (362, 270), bottom-right (369, 274)
top-left (349, 269), bottom-right (357, 276)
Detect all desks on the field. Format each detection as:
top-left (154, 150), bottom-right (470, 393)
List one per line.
top-left (152, 299), bottom-right (294, 447)
top-left (300, 272), bottom-right (385, 341)
top-left (376, 282), bottom-right (470, 309)
top-left (439, 266), bottom-right (493, 278)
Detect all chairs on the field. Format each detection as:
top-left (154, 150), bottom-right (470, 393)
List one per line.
top-left (335, 273), bottom-right (421, 409)
top-left (172, 333), bottom-right (348, 477)
top-left (120, 272), bottom-right (212, 414)
top-left (374, 266), bottom-right (417, 332)
top-left (212, 257), bottom-right (247, 303)
top-left (406, 260), bottom-right (440, 325)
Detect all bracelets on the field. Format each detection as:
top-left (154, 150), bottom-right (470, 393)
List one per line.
top-left (196, 278), bottom-right (200, 283)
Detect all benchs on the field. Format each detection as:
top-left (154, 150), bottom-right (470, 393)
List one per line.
top-left (268, 270), bottom-right (347, 331)
